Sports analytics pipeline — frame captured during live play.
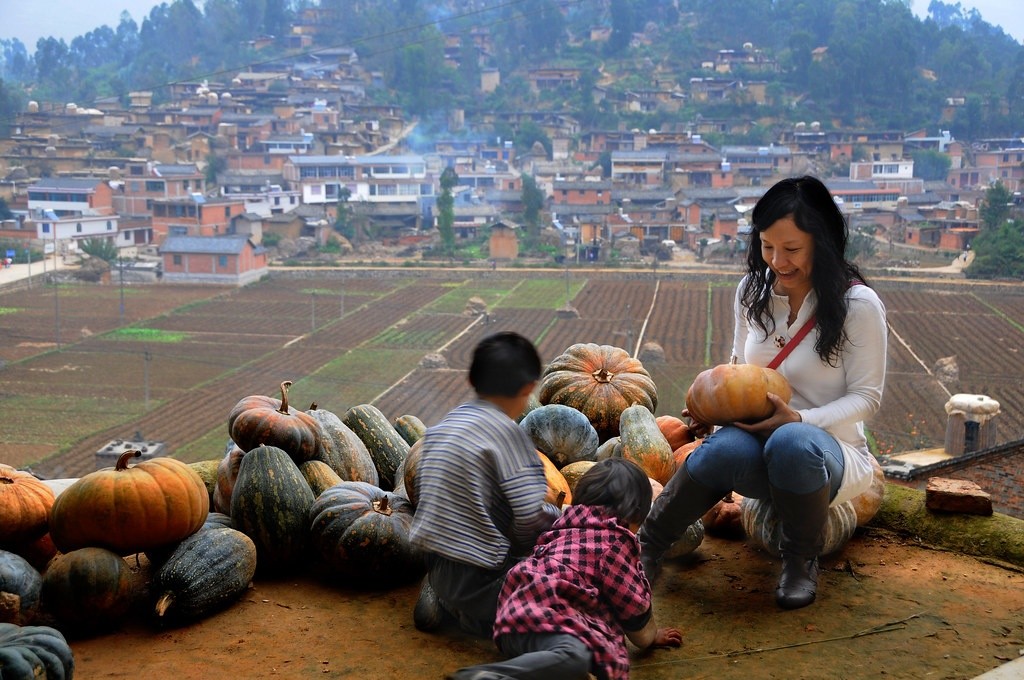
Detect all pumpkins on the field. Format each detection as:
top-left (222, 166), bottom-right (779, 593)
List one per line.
top-left (0, 380), bottom-right (428, 680)
top-left (513, 343), bottom-right (885, 556)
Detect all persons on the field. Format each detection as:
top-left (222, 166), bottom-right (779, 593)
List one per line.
top-left (407, 331), bottom-right (563, 642)
top-left (445, 458), bottom-right (683, 680)
top-left (638, 176), bottom-right (887, 607)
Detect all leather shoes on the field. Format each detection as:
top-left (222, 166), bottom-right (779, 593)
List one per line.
top-left (776, 552), bottom-right (819, 610)
top-left (638, 531), bottom-right (672, 585)
